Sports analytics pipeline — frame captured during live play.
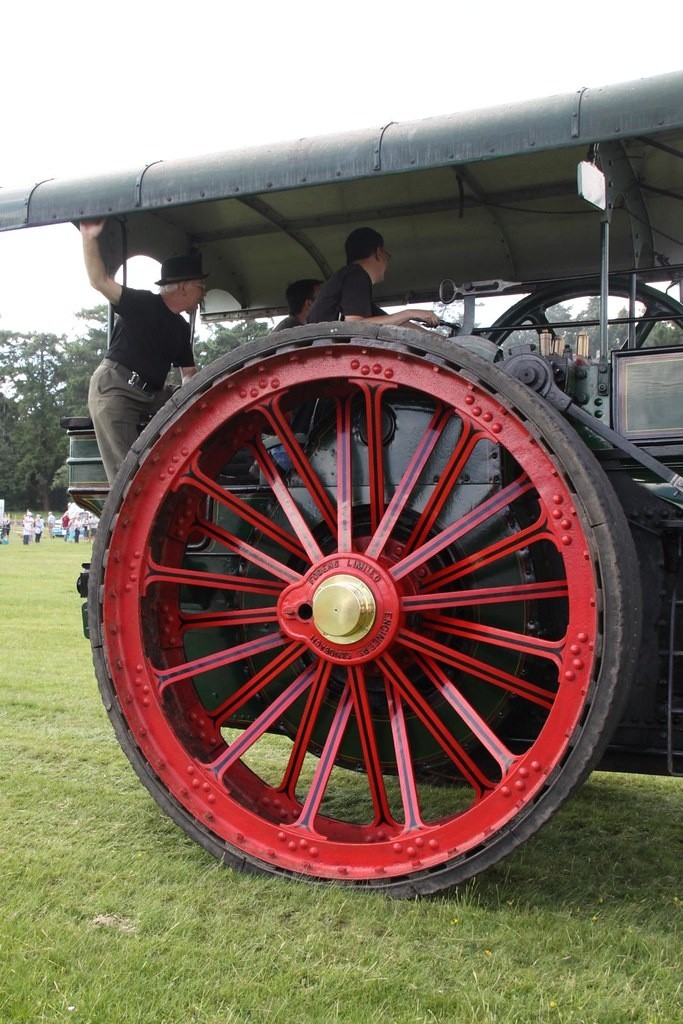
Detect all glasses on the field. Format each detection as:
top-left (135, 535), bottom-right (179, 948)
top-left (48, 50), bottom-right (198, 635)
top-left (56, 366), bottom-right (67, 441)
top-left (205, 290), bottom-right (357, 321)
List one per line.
top-left (193, 283), bottom-right (205, 292)
top-left (374, 248), bottom-right (392, 261)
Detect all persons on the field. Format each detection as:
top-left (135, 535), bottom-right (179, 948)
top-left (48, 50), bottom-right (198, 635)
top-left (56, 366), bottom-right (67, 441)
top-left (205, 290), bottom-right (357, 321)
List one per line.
top-left (248, 226), bottom-right (442, 480)
top-left (81, 217), bottom-right (211, 496)
top-left (0, 508), bottom-right (97, 544)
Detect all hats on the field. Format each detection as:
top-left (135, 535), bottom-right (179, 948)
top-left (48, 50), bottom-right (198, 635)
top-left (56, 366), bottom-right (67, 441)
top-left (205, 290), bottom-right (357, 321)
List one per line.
top-left (155, 255), bottom-right (209, 285)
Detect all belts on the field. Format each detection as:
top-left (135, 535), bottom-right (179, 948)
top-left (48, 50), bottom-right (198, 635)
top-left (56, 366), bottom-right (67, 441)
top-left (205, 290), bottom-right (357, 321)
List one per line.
top-left (102, 358), bottom-right (152, 392)
